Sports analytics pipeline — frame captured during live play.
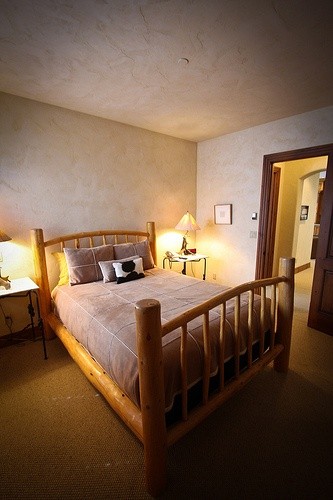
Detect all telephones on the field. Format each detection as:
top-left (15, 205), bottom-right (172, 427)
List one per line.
top-left (165, 251), bottom-right (179, 258)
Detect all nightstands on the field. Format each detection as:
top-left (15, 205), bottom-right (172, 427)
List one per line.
top-left (0, 276), bottom-right (48, 360)
top-left (162, 256), bottom-right (206, 281)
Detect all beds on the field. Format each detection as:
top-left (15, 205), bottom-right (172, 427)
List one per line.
top-left (32, 221), bottom-right (295, 500)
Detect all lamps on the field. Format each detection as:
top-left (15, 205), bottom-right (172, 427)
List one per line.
top-left (176, 211), bottom-right (199, 255)
top-left (0, 227), bottom-right (12, 289)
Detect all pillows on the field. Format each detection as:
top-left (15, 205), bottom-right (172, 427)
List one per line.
top-left (51, 240), bottom-right (155, 286)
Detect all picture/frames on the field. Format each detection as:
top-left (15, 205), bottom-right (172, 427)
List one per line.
top-left (213, 204), bottom-right (232, 224)
